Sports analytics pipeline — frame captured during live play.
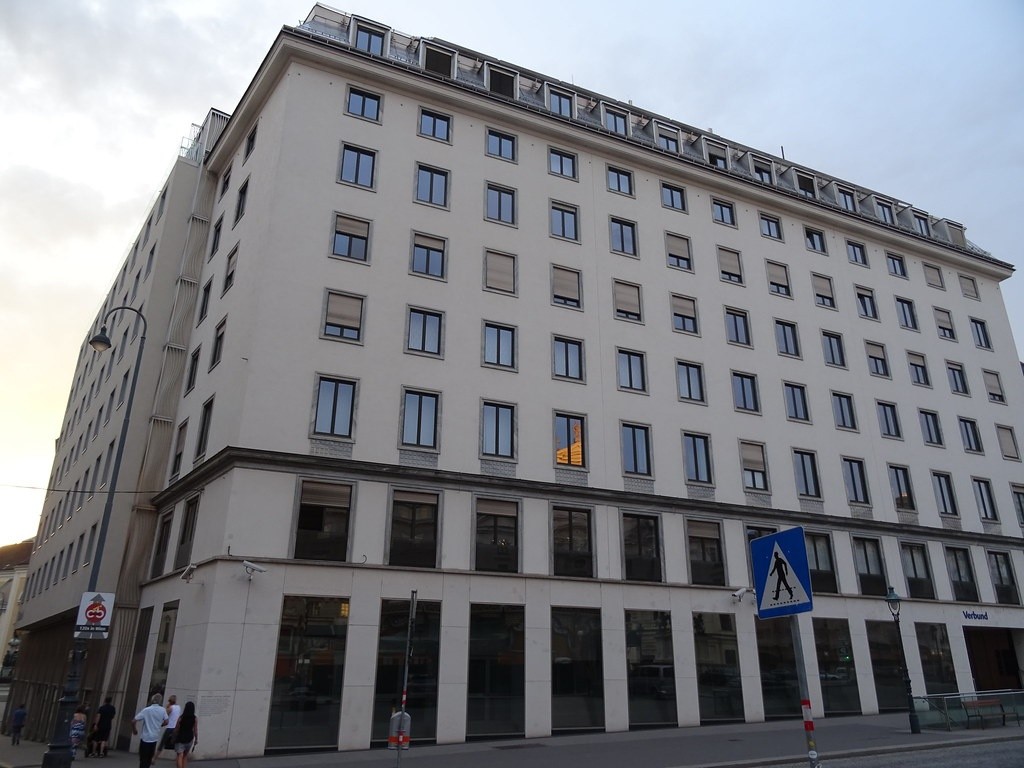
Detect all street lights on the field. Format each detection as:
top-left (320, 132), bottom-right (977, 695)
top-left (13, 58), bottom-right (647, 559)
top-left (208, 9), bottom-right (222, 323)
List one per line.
top-left (886, 585), bottom-right (921, 735)
top-left (42, 305), bottom-right (148, 768)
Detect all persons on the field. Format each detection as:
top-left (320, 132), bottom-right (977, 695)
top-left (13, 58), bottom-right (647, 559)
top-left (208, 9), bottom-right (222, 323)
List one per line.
top-left (131, 694), bottom-right (198, 767)
top-left (89, 697), bottom-right (116, 759)
top-left (11, 704), bottom-right (27, 745)
top-left (70, 705), bottom-right (88, 760)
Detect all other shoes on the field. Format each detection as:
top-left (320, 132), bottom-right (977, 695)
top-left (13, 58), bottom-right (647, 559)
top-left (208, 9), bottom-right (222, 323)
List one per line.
top-left (89, 753), bottom-right (98, 758)
top-left (151, 759), bottom-right (156, 764)
top-left (100, 753), bottom-right (104, 757)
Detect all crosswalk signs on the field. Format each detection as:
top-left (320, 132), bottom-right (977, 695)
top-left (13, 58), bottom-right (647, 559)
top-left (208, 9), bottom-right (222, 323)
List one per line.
top-left (748, 525), bottom-right (813, 620)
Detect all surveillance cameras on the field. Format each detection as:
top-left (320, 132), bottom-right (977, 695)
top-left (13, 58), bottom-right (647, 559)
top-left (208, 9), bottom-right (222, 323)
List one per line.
top-left (732, 587), bottom-right (746, 597)
top-left (243, 561), bottom-right (267, 572)
top-left (180, 562), bottom-right (197, 580)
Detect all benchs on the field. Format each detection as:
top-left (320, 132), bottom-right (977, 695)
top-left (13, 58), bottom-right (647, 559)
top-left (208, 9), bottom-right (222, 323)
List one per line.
top-left (961, 699), bottom-right (1020, 730)
